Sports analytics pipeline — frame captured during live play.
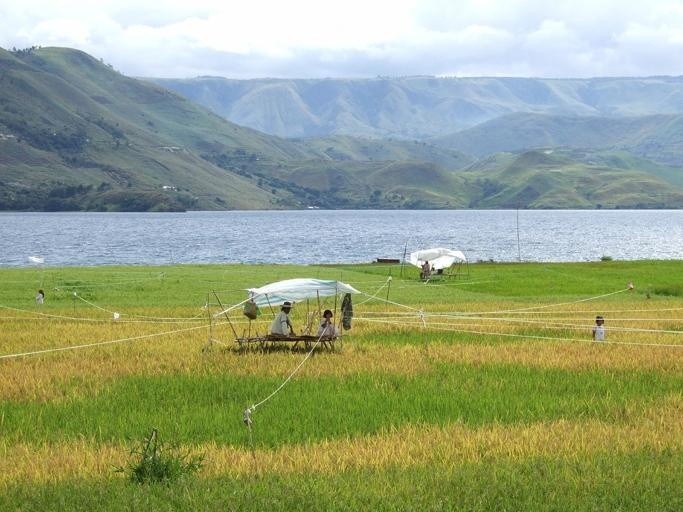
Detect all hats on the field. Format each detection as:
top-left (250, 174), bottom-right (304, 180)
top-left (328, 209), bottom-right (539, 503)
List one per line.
top-left (279, 301), bottom-right (294, 309)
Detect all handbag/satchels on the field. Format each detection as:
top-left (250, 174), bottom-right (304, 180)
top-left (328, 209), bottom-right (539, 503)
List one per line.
top-left (243, 301), bottom-right (258, 320)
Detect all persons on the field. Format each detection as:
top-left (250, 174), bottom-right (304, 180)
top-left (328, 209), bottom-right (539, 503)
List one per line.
top-left (34, 289), bottom-right (44, 305)
top-left (316, 309), bottom-right (338, 338)
top-left (591, 315), bottom-right (604, 341)
top-left (422, 260), bottom-right (430, 279)
top-left (269, 301), bottom-right (296, 336)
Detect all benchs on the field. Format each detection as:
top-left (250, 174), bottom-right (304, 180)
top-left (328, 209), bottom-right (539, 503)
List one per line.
top-left (234, 334), bottom-right (336, 352)
